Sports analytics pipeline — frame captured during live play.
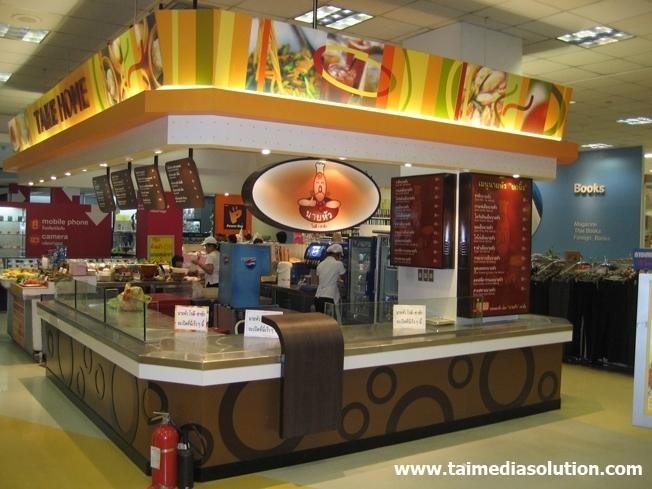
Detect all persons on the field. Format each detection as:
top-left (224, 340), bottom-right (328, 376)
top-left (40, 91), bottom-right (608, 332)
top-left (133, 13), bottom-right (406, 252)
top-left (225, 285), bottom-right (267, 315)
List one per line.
top-left (191, 236), bottom-right (221, 289)
top-left (314, 243), bottom-right (348, 314)
top-left (172, 255), bottom-right (185, 269)
top-left (228, 234), bottom-right (238, 243)
top-left (276, 231), bottom-right (287, 243)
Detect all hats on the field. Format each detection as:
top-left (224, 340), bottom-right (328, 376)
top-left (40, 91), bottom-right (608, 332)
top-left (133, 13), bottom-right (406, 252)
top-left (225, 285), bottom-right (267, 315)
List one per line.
top-left (201, 236), bottom-right (217, 246)
top-left (328, 244), bottom-right (343, 254)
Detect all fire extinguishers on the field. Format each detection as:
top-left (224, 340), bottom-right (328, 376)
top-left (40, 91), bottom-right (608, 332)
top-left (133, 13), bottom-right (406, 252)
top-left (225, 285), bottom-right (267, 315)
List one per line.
top-left (147, 411), bottom-right (193, 489)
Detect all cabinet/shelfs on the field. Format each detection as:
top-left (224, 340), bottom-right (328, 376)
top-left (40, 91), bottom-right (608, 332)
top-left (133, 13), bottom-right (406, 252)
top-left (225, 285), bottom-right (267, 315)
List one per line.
top-left (112, 209), bottom-right (137, 258)
top-left (531, 261), bottom-right (639, 375)
top-left (0, 206), bottom-right (27, 268)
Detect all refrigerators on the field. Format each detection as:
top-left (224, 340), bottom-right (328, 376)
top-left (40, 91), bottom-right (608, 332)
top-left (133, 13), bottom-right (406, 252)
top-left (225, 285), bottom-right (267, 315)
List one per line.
top-left (346, 234), bottom-right (399, 324)
